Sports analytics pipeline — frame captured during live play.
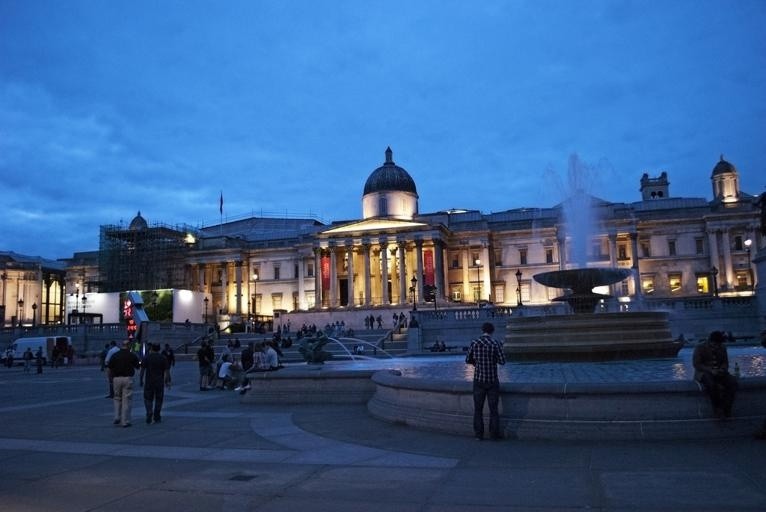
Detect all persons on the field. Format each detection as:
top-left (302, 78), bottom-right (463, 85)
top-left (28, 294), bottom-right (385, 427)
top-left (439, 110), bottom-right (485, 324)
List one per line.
top-left (200, 311), bottom-right (409, 394)
top-left (185, 318), bottom-right (192, 329)
top-left (439, 340), bottom-right (445, 352)
top-left (431, 340), bottom-right (439, 352)
top-left (6, 343), bottom-right (73, 374)
top-left (465, 322), bottom-right (506, 440)
top-left (692, 331), bottom-right (738, 419)
top-left (99, 340), bottom-right (175, 427)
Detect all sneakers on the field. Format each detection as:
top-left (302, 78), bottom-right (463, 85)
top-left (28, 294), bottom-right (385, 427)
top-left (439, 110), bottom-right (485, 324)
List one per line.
top-left (474, 432), bottom-right (482, 441)
top-left (154, 415), bottom-right (161, 422)
top-left (490, 433), bottom-right (502, 441)
top-left (146, 410), bottom-right (153, 424)
top-left (199, 382), bottom-right (252, 392)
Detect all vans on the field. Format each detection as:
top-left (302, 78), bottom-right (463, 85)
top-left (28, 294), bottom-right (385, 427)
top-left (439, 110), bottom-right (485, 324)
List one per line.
top-left (0, 336), bottom-right (72, 367)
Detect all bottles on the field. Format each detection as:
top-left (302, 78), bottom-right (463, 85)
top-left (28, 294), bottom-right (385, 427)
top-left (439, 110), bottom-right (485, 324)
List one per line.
top-left (735, 362), bottom-right (740, 379)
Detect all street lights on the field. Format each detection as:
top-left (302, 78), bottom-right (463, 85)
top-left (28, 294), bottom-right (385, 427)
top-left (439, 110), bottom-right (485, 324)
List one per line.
top-left (744, 238), bottom-right (752, 269)
top-left (81, 295), bottom-right (87, 323)
top-left (151, 289), bottom-right (158, 320)
top-left (514, 267), bottom-right (524, 306)
top-left (17, 299), bottom-right (24, 327)
top-left (516, 288), bottom-right (520, 304)
top-left (709, 265), bottom-right (719, 295)
top-left (203, 296), bottom-right (209, 324)
top-left (75, 282), bottom-right (80, 314)
top-left (32, 303), bottom-right (38, 327)
top-left (252, 274), bottom-right (258, 319)
top-left (431, 285), bottom-right (438, 312)
top-left (475, 260), bottom-right (482, 305)
top-left (411, 276), bottom-right (418, 311)
